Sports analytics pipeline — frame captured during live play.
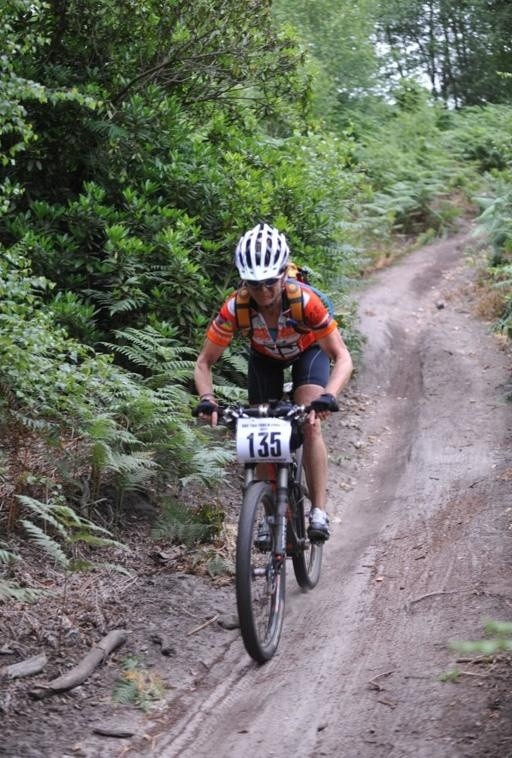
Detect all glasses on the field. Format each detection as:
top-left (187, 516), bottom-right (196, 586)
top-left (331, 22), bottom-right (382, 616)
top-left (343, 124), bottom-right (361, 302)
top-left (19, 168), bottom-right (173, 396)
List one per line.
top-left (248, 270), bottom-right (284, 288)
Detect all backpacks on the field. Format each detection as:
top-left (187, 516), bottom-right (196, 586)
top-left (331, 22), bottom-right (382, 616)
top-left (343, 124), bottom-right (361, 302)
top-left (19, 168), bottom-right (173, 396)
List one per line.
top-left (234, 262), bottom-right (307, 332)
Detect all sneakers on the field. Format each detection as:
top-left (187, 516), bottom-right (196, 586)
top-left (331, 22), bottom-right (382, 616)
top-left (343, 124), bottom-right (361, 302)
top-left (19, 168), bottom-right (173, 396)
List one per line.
top-left (307, 510), bottom-right (330, 541)
top-left (255, 526), bottom-right (272, 551)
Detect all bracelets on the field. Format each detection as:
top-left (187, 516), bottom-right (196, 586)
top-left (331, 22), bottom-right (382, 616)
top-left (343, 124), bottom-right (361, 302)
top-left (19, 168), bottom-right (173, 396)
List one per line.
top-left (200, 393), bottom-right (217, 400)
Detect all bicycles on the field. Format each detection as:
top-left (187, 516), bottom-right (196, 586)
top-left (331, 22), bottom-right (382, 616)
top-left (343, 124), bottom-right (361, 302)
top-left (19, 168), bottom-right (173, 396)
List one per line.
top-left (190, 381), bottom-right (339, 661)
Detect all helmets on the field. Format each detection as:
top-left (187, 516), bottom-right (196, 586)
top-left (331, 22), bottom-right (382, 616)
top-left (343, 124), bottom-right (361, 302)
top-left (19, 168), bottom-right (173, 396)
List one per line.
top-left (235, 223), bottom-right (289, 280)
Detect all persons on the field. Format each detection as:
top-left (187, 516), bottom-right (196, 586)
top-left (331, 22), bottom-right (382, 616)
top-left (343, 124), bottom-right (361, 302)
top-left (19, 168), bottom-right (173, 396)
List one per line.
top-left (194, 221), bottom-right (354, 548)
